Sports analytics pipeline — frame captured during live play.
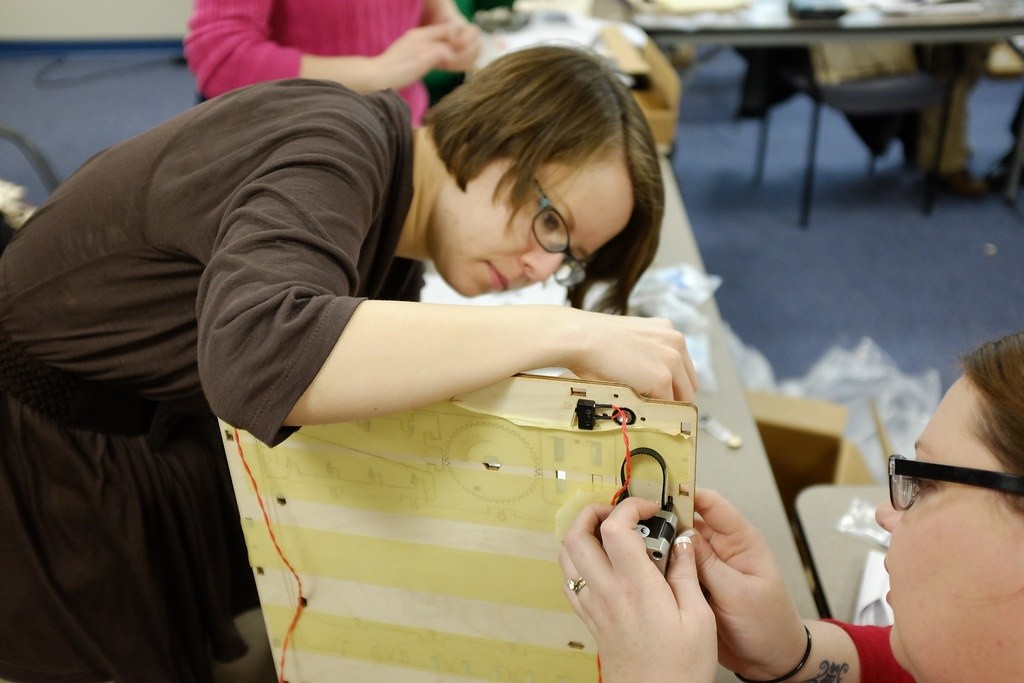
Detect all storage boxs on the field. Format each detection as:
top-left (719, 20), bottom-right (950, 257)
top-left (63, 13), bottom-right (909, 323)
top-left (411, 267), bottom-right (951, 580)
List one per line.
top-left (741, 384), bottom-right (881, 593)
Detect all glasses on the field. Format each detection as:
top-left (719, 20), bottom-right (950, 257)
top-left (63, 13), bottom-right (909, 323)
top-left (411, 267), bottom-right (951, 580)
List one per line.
top-left (888, 454), bottom-right (1024, 510)
top-left (529, 171), bottom-right (589, 286)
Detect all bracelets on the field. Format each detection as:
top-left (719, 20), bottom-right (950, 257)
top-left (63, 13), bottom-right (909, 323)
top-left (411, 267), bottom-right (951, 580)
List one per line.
top-left (735, 625), bottom-right (812, 683)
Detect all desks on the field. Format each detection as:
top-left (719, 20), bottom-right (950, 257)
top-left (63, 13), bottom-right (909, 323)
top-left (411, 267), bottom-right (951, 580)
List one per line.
top-left (635, 0), bottom-right (1024, 206)
top-left (636, 156), bottom-right (820, 683)
top-left (795, 484), bottom-right (890, 623)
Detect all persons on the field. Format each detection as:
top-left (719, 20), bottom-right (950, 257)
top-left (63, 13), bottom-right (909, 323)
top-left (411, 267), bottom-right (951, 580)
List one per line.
top-left (560, 334), bottom-right (1024, 683)
top-left (183, 0), bottom-right (485, 126)
top-left (0, 47), bottom-right (696, 683)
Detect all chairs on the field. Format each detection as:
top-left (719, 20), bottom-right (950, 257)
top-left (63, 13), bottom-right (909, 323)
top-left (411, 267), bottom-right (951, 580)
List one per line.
top-left (752, 42), bottom-right (959, 228)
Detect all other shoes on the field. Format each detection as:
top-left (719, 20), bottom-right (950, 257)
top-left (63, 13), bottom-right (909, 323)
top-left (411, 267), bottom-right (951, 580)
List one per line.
top-left (936, 171), bottom-right (980, 203)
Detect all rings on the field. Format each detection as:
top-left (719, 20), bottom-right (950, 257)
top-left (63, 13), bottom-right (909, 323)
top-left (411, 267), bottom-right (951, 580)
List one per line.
top-left (567, 578), bottom-right (586, 595)
